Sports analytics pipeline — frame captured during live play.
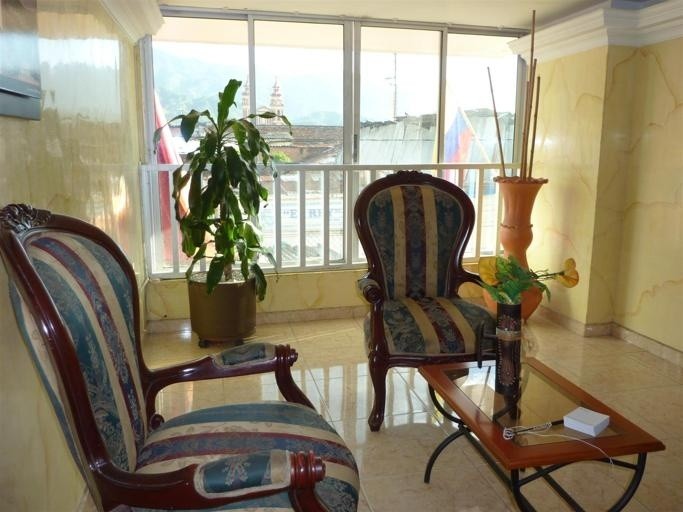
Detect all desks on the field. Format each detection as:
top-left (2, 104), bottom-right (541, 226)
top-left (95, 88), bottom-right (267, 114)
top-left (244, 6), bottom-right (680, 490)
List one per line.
top-left (418, 357), bottom-right (665, 512)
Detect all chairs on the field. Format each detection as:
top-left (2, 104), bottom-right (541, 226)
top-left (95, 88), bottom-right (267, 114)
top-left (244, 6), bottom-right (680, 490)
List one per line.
top-left (351, 168), bottom-right (506, 432)
top-left (0, 200), bottom-right (359, 512)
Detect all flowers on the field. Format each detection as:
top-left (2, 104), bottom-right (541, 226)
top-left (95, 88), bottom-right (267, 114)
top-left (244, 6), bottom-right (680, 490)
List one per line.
top-left (485, 256), bottom-right (580, 309)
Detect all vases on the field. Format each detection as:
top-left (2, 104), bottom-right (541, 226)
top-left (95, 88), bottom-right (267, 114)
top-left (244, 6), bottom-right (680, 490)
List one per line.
top-left (492, 303), bottom-right (523, 395)
top-left (480, 175), bottom-right (546, 320)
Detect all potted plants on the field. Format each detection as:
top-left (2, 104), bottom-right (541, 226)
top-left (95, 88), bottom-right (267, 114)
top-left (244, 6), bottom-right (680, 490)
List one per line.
top-left (150, 79), bottom-right (295, 351)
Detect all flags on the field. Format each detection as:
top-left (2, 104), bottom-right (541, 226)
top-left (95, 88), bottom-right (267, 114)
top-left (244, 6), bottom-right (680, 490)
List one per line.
top-left (150, 95), bottom-right (216, 266)
top-left (428, 100), bottom-right (474, 184)
